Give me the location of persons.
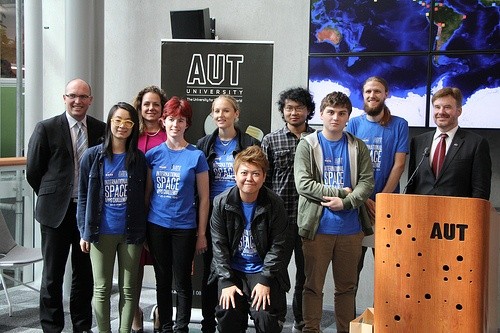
[261,88,316,333]
[145,96,209,333]
[197,94,261,333]
[346,76,409,333]
[77,102,146,333]
[131,85,166,333]
[26,79,107,333]
[294,91,375,333]
[209,145,294,333]
[405,88,492,201]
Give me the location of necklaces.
[219,132,235,155]
[145,128,160,136]
[167,143,187,169]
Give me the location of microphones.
[402,146,430,194]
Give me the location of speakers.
[170,7,216,39]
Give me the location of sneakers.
[292,320,303,333]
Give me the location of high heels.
[130,311,144,333]
[153,306,160,333]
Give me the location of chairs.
[0,209,43,316]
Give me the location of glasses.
[111,119,134,129]
[284,105,306,112]
[65,94,89,98]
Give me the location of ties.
[432,133,448,180]
[75,122,88,198]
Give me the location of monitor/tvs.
[307,0,500,131]
